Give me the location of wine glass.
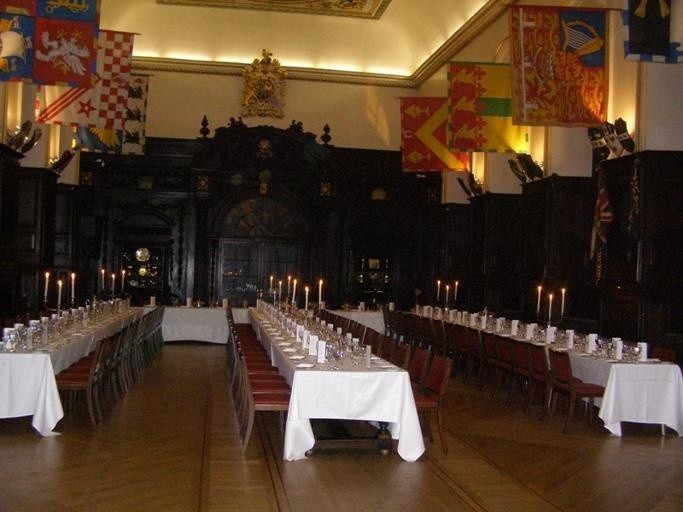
[3,297,131,353]
[268,300,367,370]
[412,304,652,362]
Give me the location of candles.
[99,269,125,293]
[435,279,458,305]
[268,275,323,311]
[40,272,76,307]
[536,286,564,320]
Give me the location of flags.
[445,59,532,156]
[507,3,611,130]
[70,73,150,157]
[399,96,472,174]
[625,171,643,240]
[31,30,134,132]
[1,0,102,89]
[586,175,616,259]
[619,0,683,64]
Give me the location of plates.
[34,307,142,354]
[250,306,400,370]
[413,309,615,364]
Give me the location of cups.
[150,297,394,311]
